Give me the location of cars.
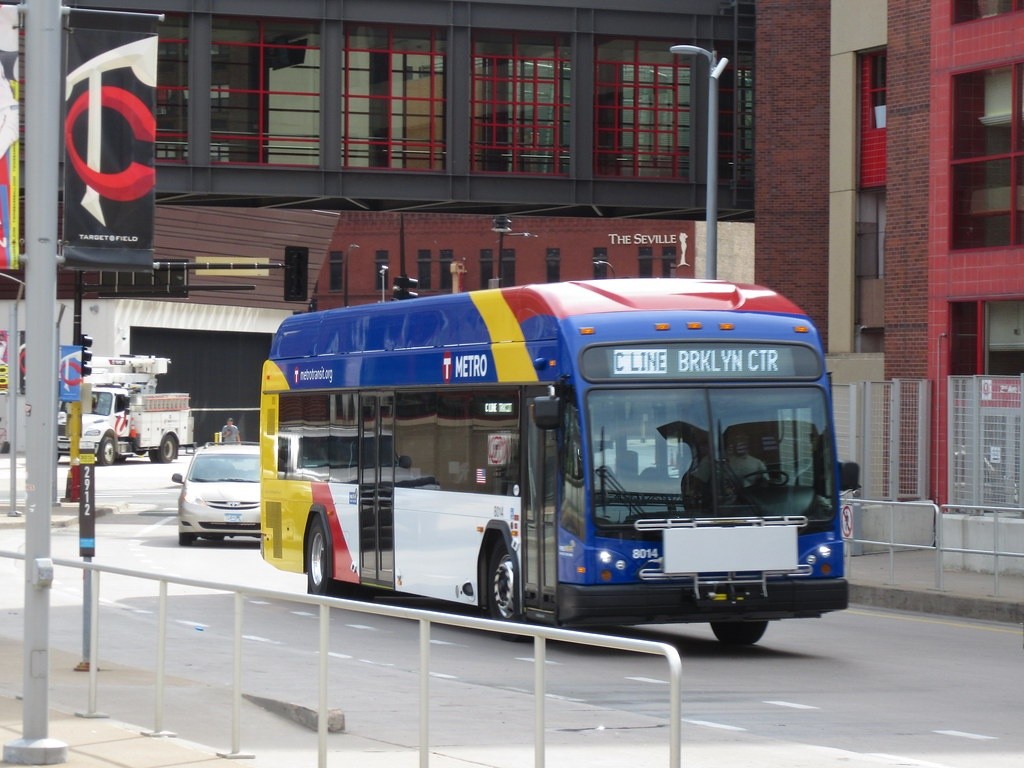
[171,441,261,546]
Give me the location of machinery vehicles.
[58,355,195,466]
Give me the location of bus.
[258,283,864,646]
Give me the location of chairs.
[627,450,656,479]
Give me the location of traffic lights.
[392,276,420,300]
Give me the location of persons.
[220,418,241,445]
[719,434,770,504]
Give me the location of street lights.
[491,216,514,288]
[669,40,729,279]
[592,259,617,279]
